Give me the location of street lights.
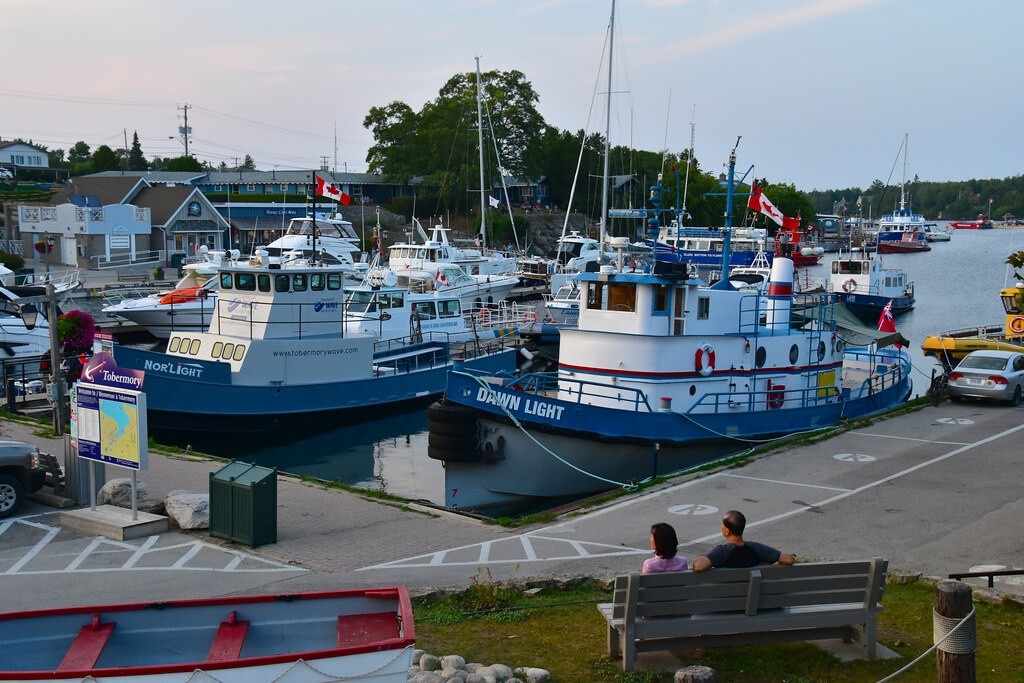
[361,200,368,253]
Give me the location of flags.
[747,181,799,231]
[314,176,351,208]
[436,271,449,287]
[489,195,499,209]
[877,300,901,349]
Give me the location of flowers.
[57,309,96,349]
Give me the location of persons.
[693,510,796,572]
[529,199,536,211]
[521,196,529,213]
[536,200,540,214]
[501,204,508,215]
[642,522,688,574]
[629,258,642,273]
[503,243,513,258]
[308,252,319,266]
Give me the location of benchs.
[373,347,444,376]
[595,557,889,672]
[115,266,149,282]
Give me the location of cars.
[946,350,1024,406]
[0,439,47,518]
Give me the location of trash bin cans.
[171,253,186,268]
[63,433,106,509]
[209,459,278,551]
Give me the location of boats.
[0,1,952,517]
[920,234,1024,375]
[948,198,995,230]
[0,582,418,683]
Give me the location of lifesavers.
[479,308,491,322]
[695,343,715,377]
[1009,316,1024,334]
[841,278,857,293]
[428,401,482,463]
